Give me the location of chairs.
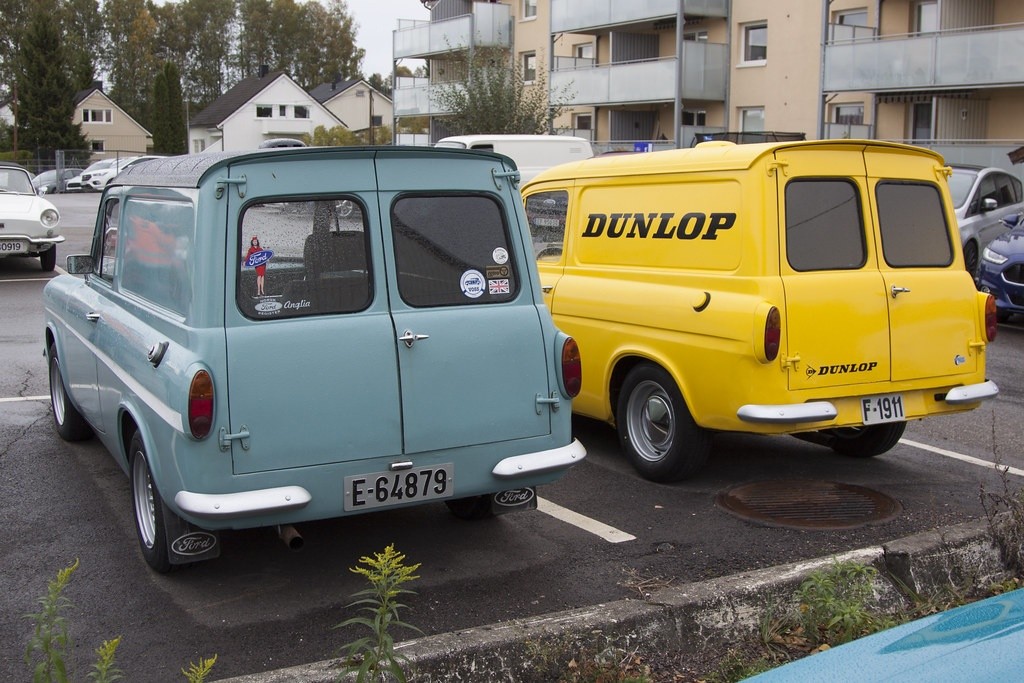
[303,229,367,274]
[282,277,369,319]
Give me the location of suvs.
[40,137,589,577]
[526,141,1000,483]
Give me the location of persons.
[131,212,190,299]
[247,238,267,297]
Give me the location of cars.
[0,161,65,271]
[91,154,168,193]
[975,212,1024,331]
[31,167,86,196]
[65,156,129,192]
[944,161,1024,277]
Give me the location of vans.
[432,133,596,195]
